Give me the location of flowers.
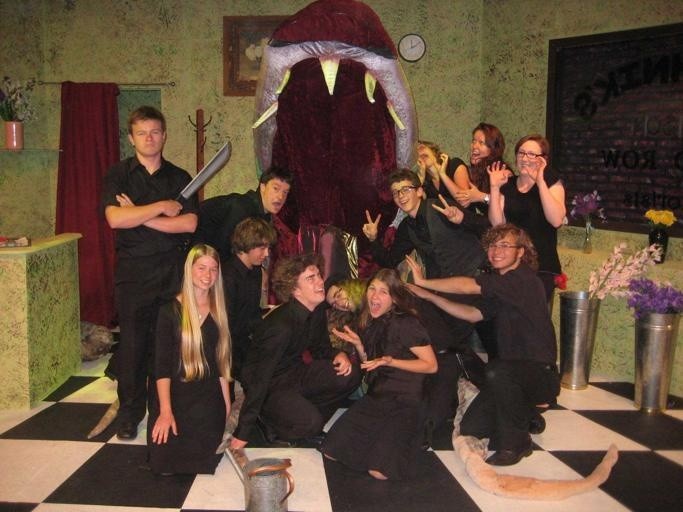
[644,206,676,225]
[586,243,662,303]
[567,190,609,240]
[553,272,567,294]
[622,274,681,314]
[0,75,37,121]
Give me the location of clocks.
[395,33,427,63]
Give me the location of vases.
[5,119,24,151]
[580,218,592,254]
[649,224,668,263]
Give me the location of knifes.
[174,141,232,205]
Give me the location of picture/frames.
[221,14,293,98]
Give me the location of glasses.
[391,185,417,197]
[489,243,520,251]
[516,151,544,159]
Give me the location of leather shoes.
[485,448,532,465]
[117,422,137,438]
[529,414,545,434]
[256,415,295,448]
